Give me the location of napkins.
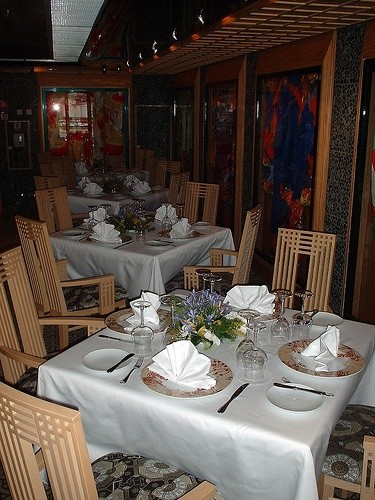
[127,289,161,331]
[154,203,176,227]
[77,177,91,188]
[301,325,341,374]
[83,183,105,197]
[90,221,122,245]
[224,283,277,314]
[147,341,217,392]
[134,181,150,195]
[124,175,141,186]
[83,208,110,223]
[169,217,192,239]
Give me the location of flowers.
[168,291,250,350]
[108,209,150,233]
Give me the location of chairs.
[0,148,375,500]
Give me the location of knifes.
[273,382,334,398]
[107,353,135,373]
[113,241,134,250]
[217,383,249,414]
[120,356,144,385]
[99,334,133,343]
[154,239,174,244]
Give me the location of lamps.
[117,63,121,72]
[101,64,107,74]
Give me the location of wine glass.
[176,203,186,222]
[195,268,212,291]
[131,301,154,358]
[243,322,269,381]
[159,294,183,347]
[270,289,293,346]
[87,197,147,241]
[235,309,260,379]
[290,290,313,340]
[160,203,172,231]
[202,273,222,293]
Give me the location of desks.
[77,169,141,184]
[33,289,375,500]
[66,183,169,218]
[49,213,237,300]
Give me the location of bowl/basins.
[193,221,211,230]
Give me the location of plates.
[104,307,172,335]
[277,338,365,378]
[305,312,344,327]
[80,348,133,372]
[265,383,324,413]
[140,356,234,400]
[144,240,171,248]
[62,227,85,239]
